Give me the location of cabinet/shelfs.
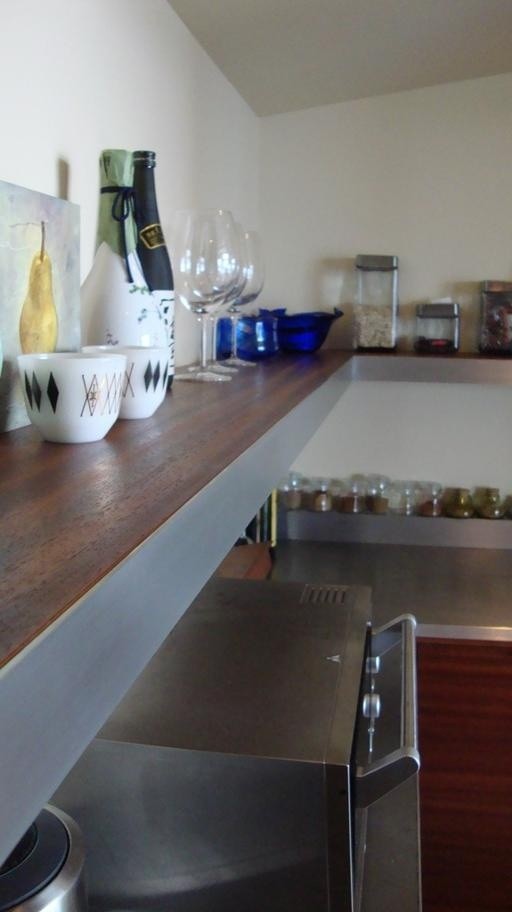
[353,340,510,394]
[0,349,354,872]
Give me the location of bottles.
[351,254,512,356]
[280,474,511,518]
[80,149,174,396]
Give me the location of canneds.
[478,280,512,356]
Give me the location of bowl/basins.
[275,308,345,352]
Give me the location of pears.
[19,222,59,354]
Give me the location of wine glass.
[178,212,266,384]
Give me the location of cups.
[17,344,171,443]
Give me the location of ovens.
[54,575,418,910]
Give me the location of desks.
[267,507,511,646]
